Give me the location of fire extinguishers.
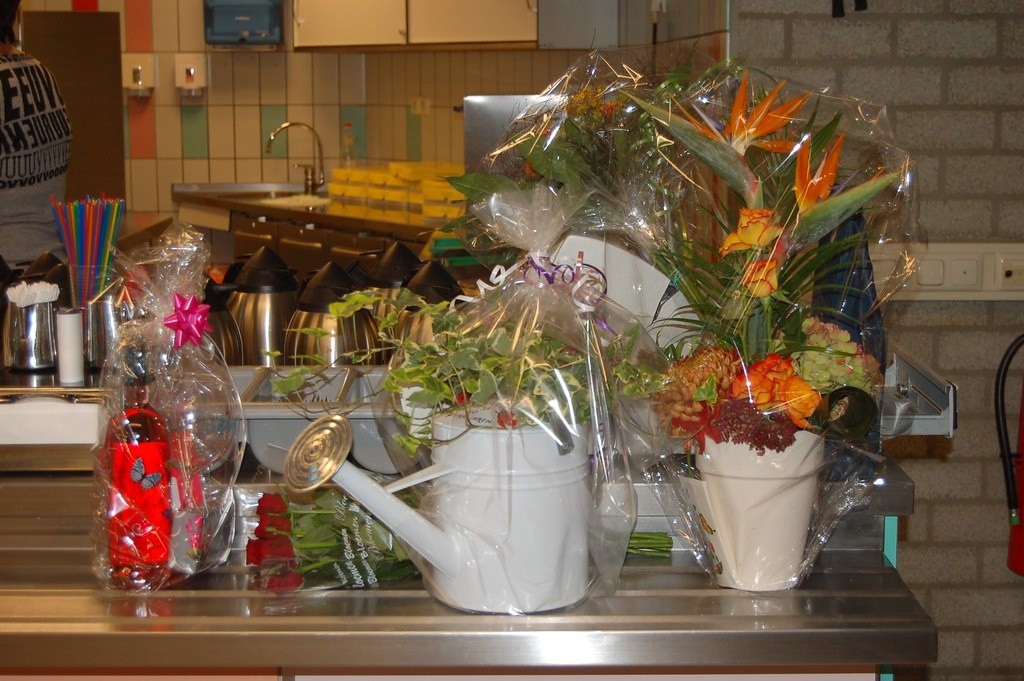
[994,331,1024,574]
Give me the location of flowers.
[242,491,673,591]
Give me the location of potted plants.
[458,43,923,594]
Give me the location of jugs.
[0,237,468,384]
[281,394,590,613]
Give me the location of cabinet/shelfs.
[290,0,540,50]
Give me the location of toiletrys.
[172,52,212,108]
[121,51,159,108]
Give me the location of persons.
[0,0,73,323]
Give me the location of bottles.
[103,325,174,588]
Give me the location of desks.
[0,472,939,680]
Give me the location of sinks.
[218,188,319,203]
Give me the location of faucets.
[266,120,326,196]
